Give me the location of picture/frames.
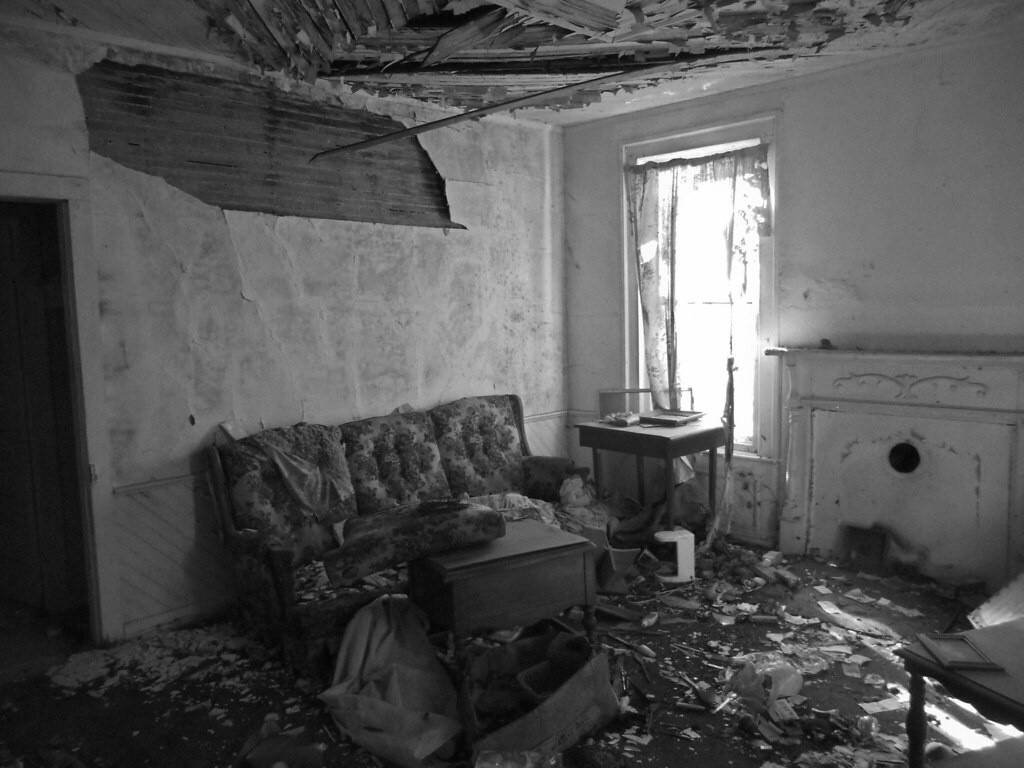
[915,632,1003,671]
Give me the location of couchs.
[203,394,588,663]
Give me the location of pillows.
[339,494,508,587]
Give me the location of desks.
[895,617,1024,768]
[577,418,726,527]
[408,519,601,717]
